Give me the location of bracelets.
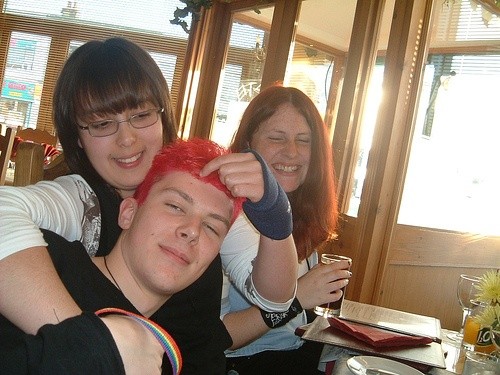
[260,296,303,329]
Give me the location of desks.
[294,314,500,375]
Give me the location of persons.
[0,37,352,375]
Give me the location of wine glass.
[448,274,485,343]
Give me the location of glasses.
[75,109,164,137]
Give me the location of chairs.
[0,125,61,187]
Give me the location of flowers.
[476,269,500,331]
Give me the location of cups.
[461,351,500,375]
[461,301,496,363]
[310,253,353,318]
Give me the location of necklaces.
[104,256,121,290]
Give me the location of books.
[294,298,449,374]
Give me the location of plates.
[345,356,424,375]
